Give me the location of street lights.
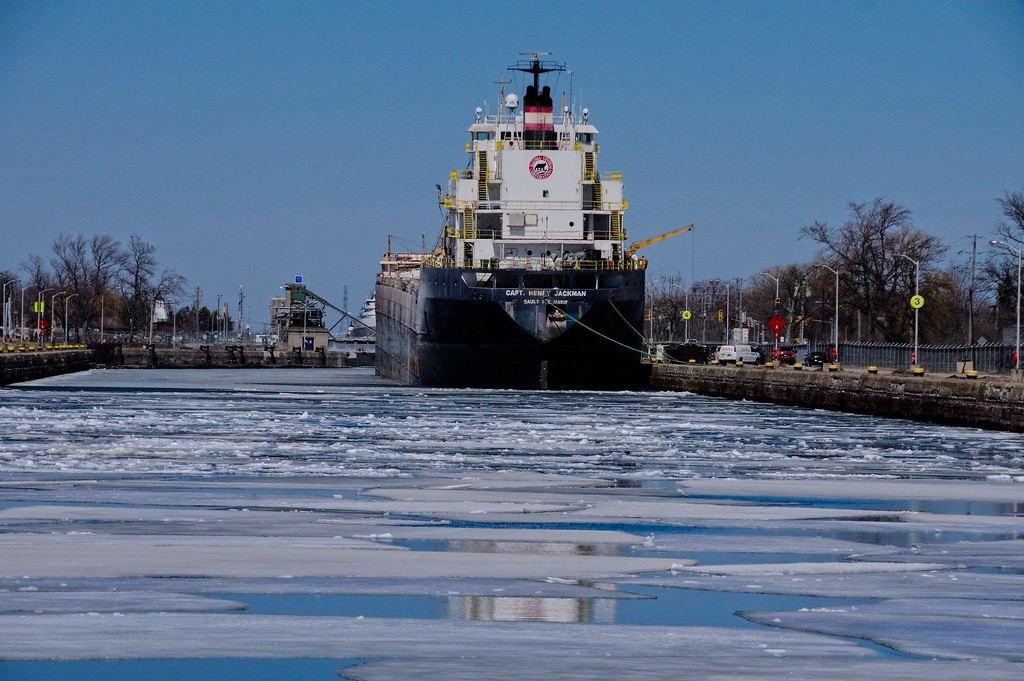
[989,239,1021,369]
[756,272,778,349]
[21,284,38,342]
[3,279,21,343]
[38,289,54,343]
[216,294,223,335]
[670,284,687,339]
[891,254,919,365]
[815,264,838,363]
[101,287,121,340]
[65,294,80,342]
[710,280,729,345]
[51,292,66,345]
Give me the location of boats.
[375,52,695,392]
[346,290,376,337]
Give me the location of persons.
[110,332,155,342]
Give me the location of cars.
[805,352,829,367]
[680,339,700,346]
[0,326,182,342]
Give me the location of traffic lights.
[766,314,788,338]
[39,319,49,330]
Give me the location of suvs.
[771,346,795,365]
[713,345,765,365]
